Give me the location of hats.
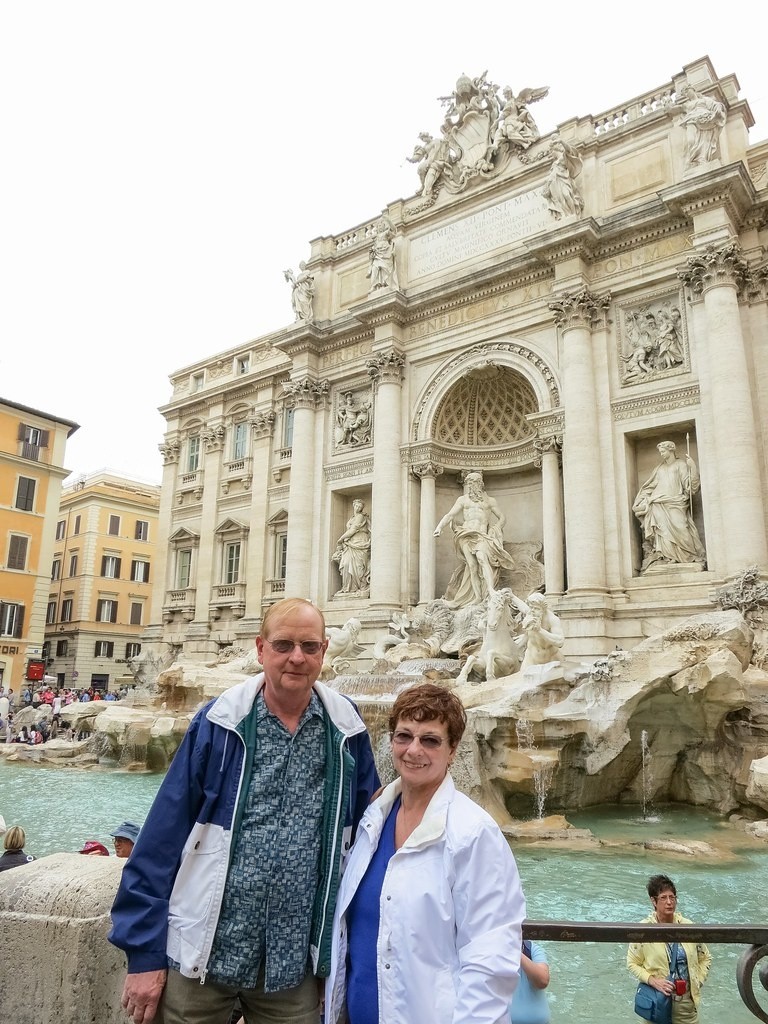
[109,821,141,844]
[79,841,109,857]
[35,689,43,693]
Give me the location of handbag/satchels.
[44,731,50,736]
[635,982,672,1024]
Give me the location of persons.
[0,712,72,745]
[491,86,531,148]
[409,131,448,196]
[500,587,564,670]
[337,392,371,447]
[0,684,121,716]
[544,139,584,220]
[366,216,399,293]
[664,84,727,170]
[434,471,506,604]
[76,840,109,856]
[110,821,140,857]
[106,597,381,1024]
[324,683,525,1024]
[337,499,371,593]
[620,312,684,385]
[626,874,712,1024]
[633,440,704,568]
[0,825,37,872]
[291,261,315,319]
[322,618,362,683]
[509,940,551,1024]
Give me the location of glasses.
[389,731,450,749]
[112,838,129,845]
[267,639,325,654]
[654,894,677,901]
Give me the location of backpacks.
[34,732,43,742]
